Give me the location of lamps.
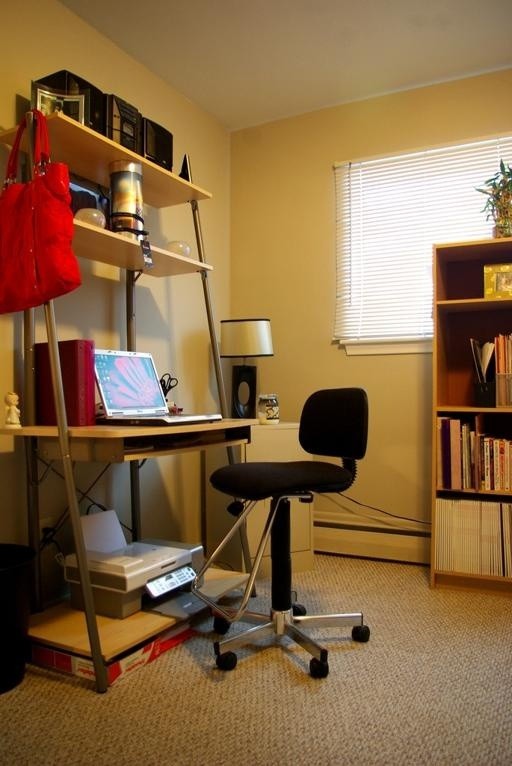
[220,318,274,418]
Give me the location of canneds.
[257,394,280,425]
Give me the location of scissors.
[160,373,178,397]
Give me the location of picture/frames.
[483,263,512,298]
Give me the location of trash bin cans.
[0,543,34,695]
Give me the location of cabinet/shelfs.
[429,239,512,598]
[2,109,257,694]
[204,418,315,584]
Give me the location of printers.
[64,538,218,620]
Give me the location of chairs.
[209,388,370,678]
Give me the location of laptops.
[94,349,222,425]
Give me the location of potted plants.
[472,159,512,237]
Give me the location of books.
[433,330,511,582]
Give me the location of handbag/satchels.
[0,108,81,315]
